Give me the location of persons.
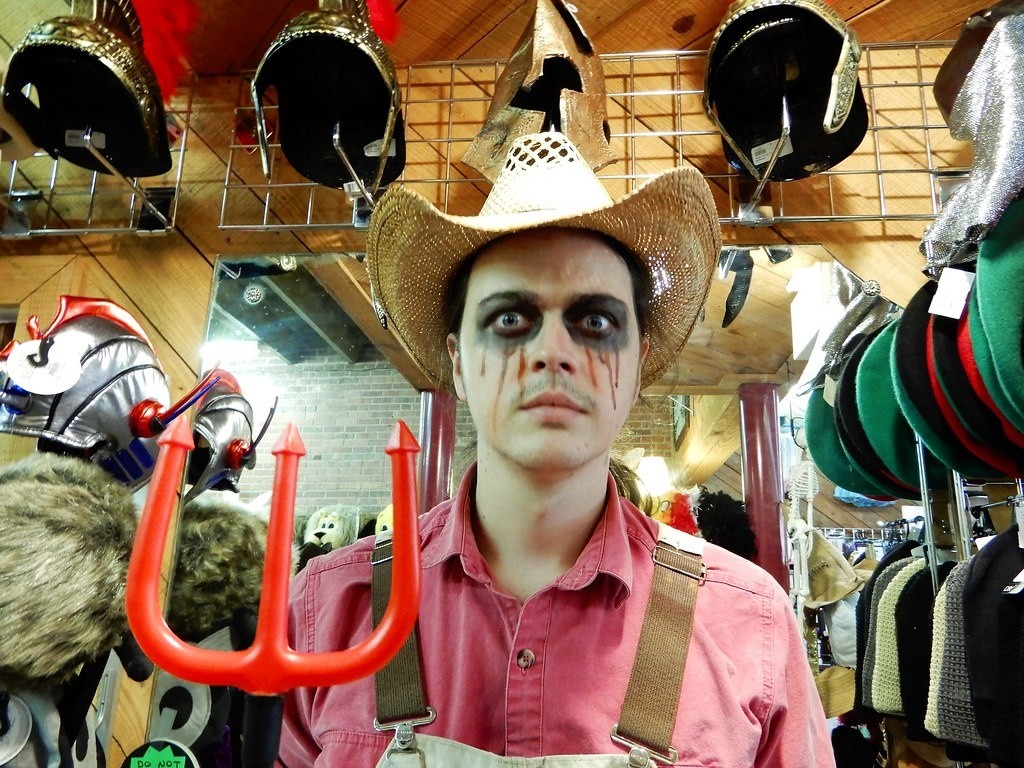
[274,133,837,767]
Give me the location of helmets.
[700,0,869,186]
[1,0,172,178]
[251,0,407,188]
[0,295,222,497]
[183,368,278,507]
[459,0,618,187]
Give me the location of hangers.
[886,515,925,547]
[970,506,995,534]
[854,531,878,544]
[1006,496,1017,524]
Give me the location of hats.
[786,201,1024,768]
[365,132,723,392]
[921,0,1024,274]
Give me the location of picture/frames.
[672,396,689,451]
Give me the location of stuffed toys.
[293,505,395,570]
